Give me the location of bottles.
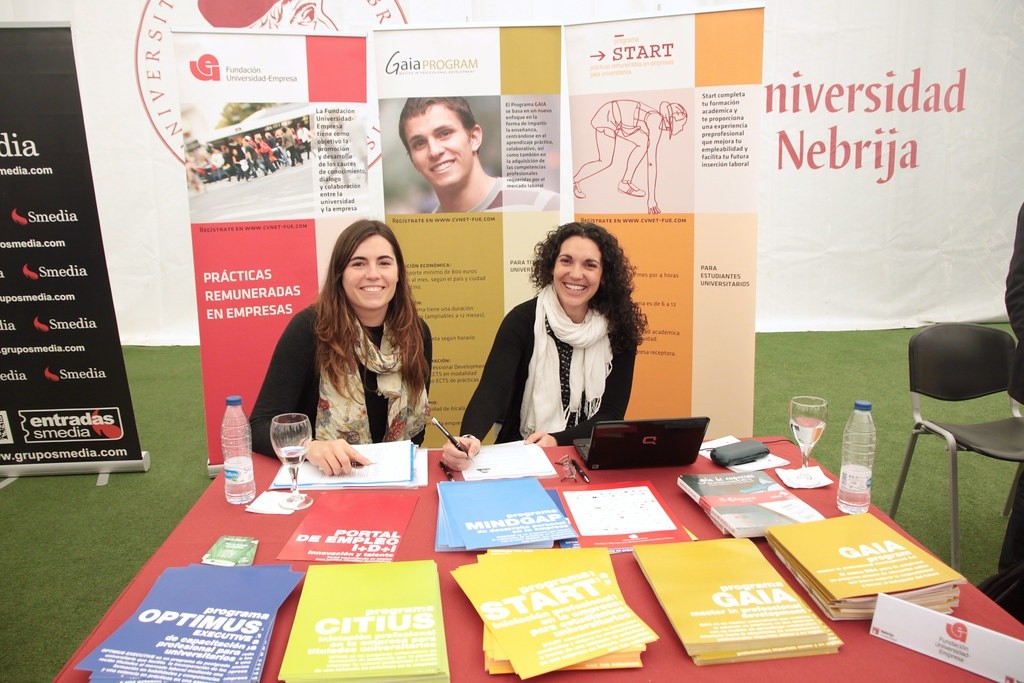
[836,400,876,514]
[220,395,256,505]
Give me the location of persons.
[399,96,561,213]
[442,221,647,471]
[186,123,310,193]
[246,219,432,474]
[997,203,1024,572]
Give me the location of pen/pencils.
[438,459,455,481]
[352,461,378,467]
[430,417,476,465]
[571,458,590,483]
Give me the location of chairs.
[889,323,1024,573]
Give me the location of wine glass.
[787,396,828,486]
[269,413,314,511]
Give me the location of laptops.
[572,416,710,470]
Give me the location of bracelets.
[301,437,309,446]
[462,434,476,439]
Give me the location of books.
[676,470,825,538]
[72,477,846,683]
[269,441,429,491]
[764,513,966,621]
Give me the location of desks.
[53,435,1024,683]
